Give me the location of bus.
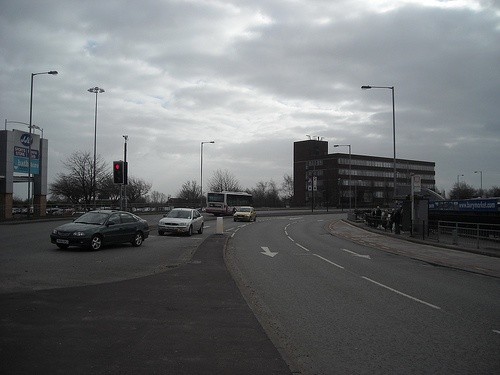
[204,191,252,217]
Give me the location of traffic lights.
[113,160,124,185]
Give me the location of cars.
[50,209,150,251]
[233,207,257,223]
[12,207,110,223]
[158,208,204,236]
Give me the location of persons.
[375,205,401,234]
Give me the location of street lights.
[201,141,215,212]
[457,174,464,188]
[122,134,129,212]
[88,87,105,205]
[26,70,59,220]
[474,170,483,190]
[333,144,352,209]
[361,84,397,209]
